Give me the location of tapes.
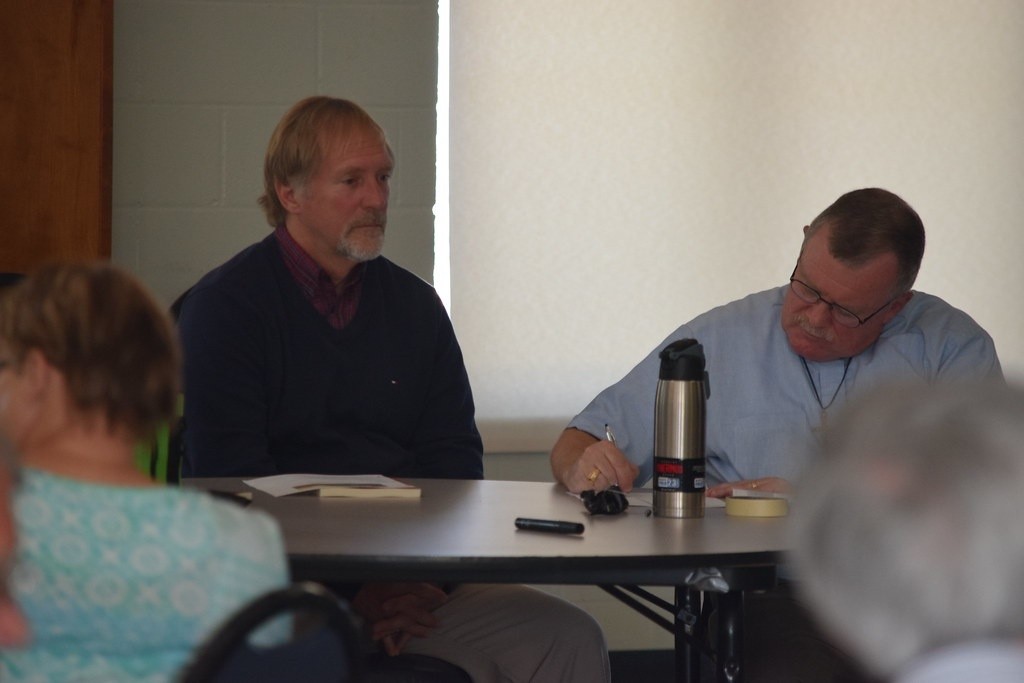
[727,496,789,518]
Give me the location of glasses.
[790,265,899,330]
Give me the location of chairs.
[180,580,362,683]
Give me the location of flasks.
[653,337,710,519]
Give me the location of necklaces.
[801,357,852,411]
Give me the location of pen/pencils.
[604,423,618,450]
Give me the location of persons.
[551,187,1006,683]
[0,261,293,683]
[151,95,611,683]
[783,373,1024,683]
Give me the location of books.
[319,475,421,498]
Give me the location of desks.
[173,476,807,683]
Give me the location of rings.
[587,468,600,482]
[752,482,757,488]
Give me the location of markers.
[514,517,585,535]
